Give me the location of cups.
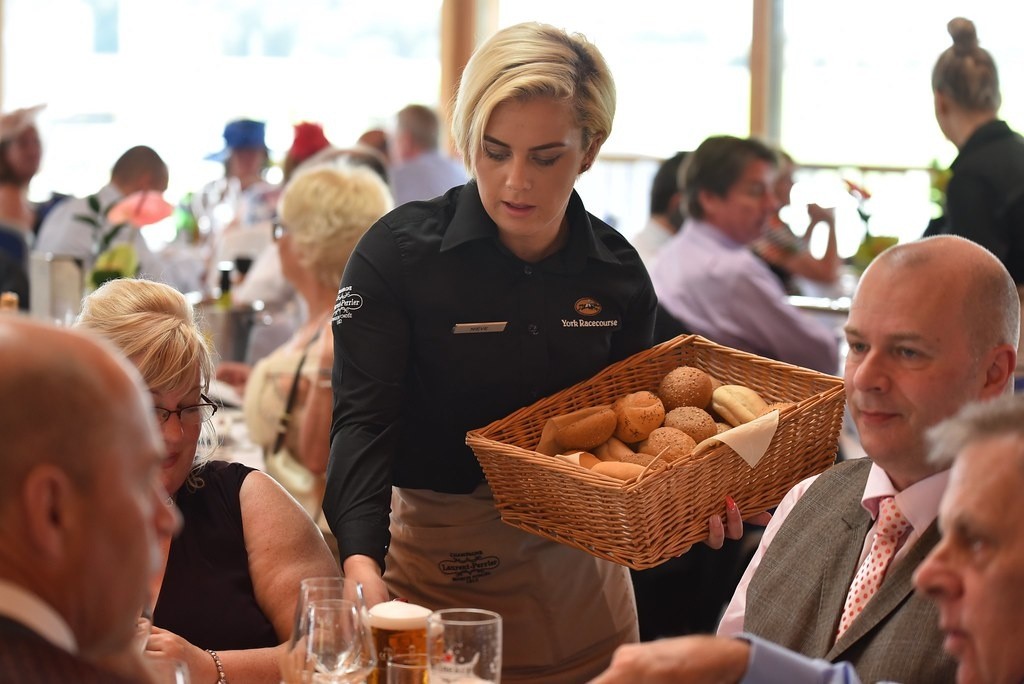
[294,599,377,684]
[367,602,435,684]
[139,657,189,684]
[279,577,371,683]
[386,654,429,684]
[428,608,502,684]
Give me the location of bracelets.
[206,649,225,684]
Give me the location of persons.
[0,316,183,684]
[631,135,838,377]
[319,20,771,684]
[588,392,1024,684]
[78,279,340,684]
[0,104,470,565]
[718,234,1024,684]
[924,18,1024,379]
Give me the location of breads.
[555,366,797,480]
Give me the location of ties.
[834,493,914,646]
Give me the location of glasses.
[272,219,294,242]
[152,394,219,427]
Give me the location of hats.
[205,119,265,160]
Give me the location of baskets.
[465,333,847,570]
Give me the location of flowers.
[73,184,171,294]
